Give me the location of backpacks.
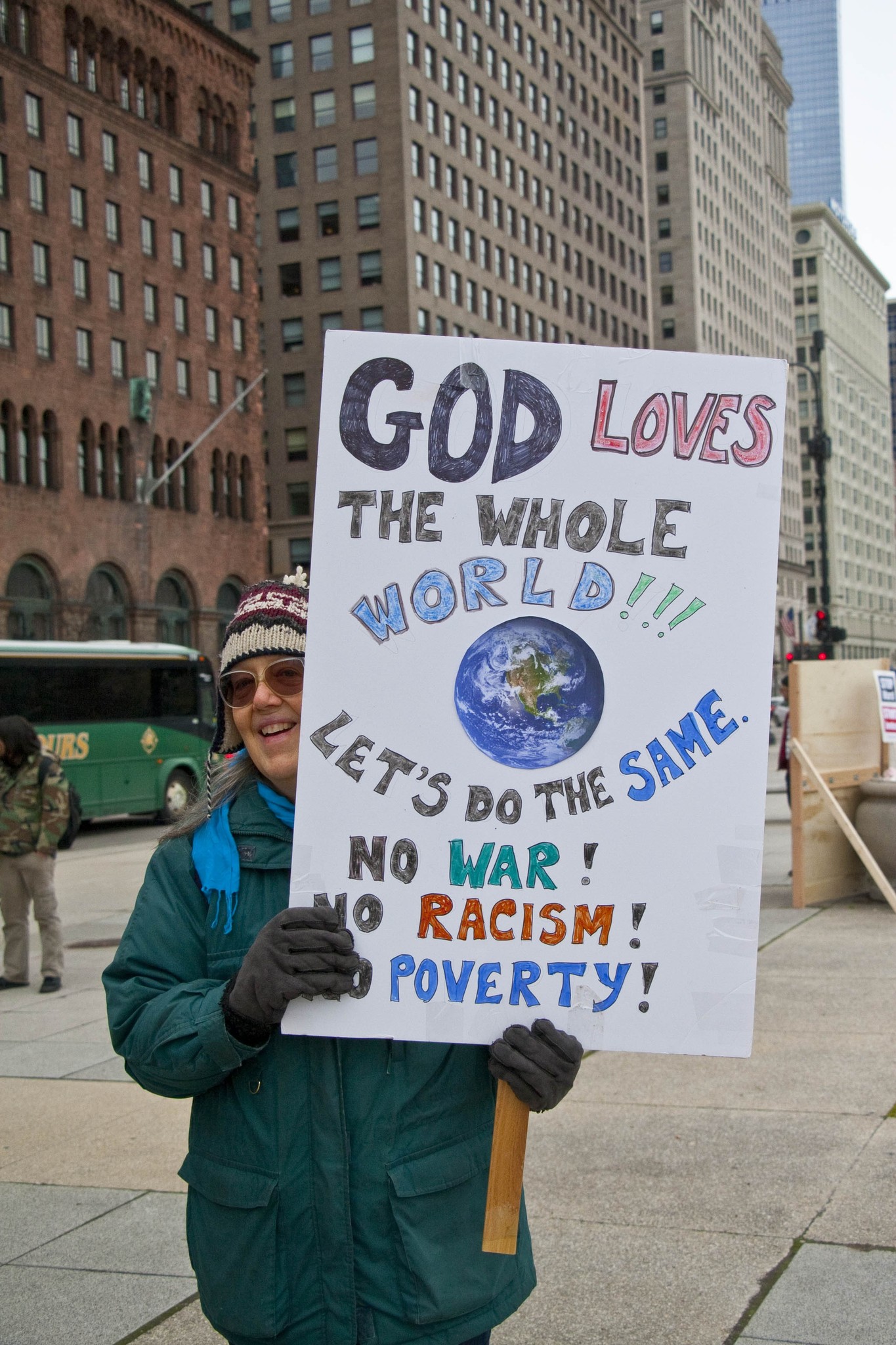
[36,758,82,850]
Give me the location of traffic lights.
[786,608,833,661]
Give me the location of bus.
[0,635,218,827]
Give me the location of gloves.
[228,906,362,1025]
[490,1018,584,1113]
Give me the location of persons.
[0,716,81,996]
[100,566,583,1345]
[777,675,793,878]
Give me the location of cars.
[770,696,790,727]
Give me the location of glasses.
[217,657,306,709]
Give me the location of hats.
[206,566,310,818]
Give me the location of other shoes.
[0,976,29,990]
[40,976,61,991]
